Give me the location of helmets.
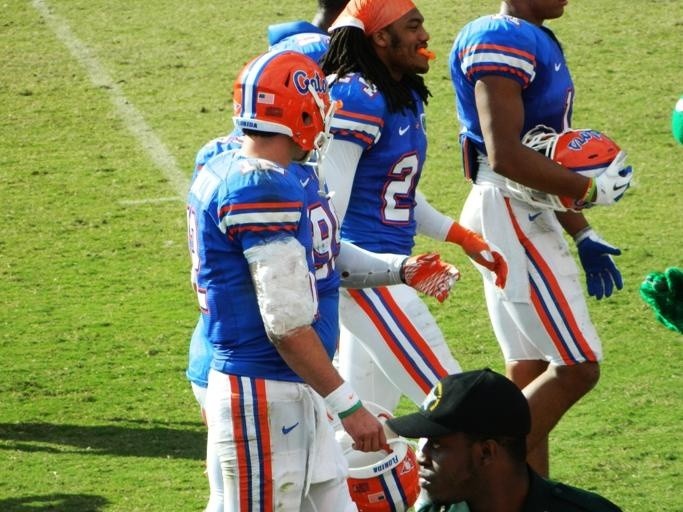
[230,48,337,167]
[507,126,624,213]
[339,432,420,511]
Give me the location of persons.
[189,0,508,511]
[386,367,623,512]
[452,1,632,478]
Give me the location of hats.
[388,367,532,440]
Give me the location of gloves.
[445,223,508,289]
[574,231,624,298]
[401,252,460,302]
[588,148,632,206]
